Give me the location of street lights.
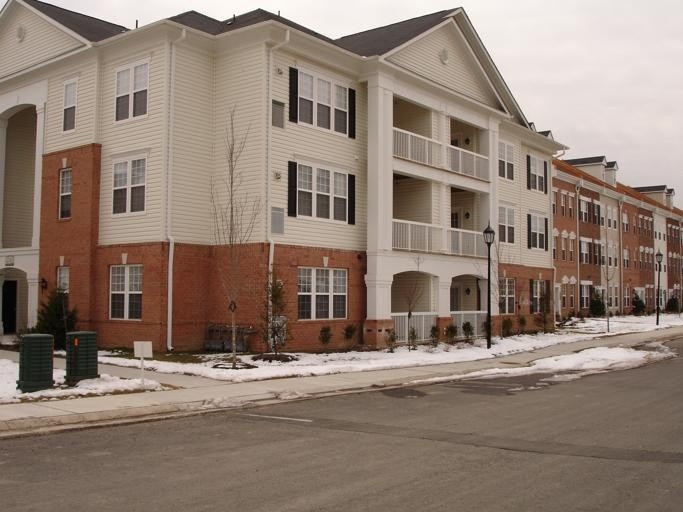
[479,219,498,351]
[653,247,665,325]
